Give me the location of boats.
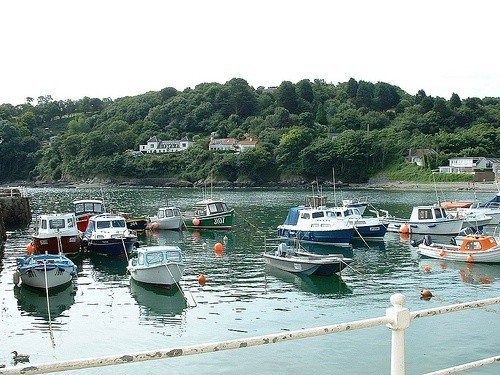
[126,246,187,286]
[263,232,353,277]
[30,170,236,256]
[16,250,77,290]
[275,166,500,263]
